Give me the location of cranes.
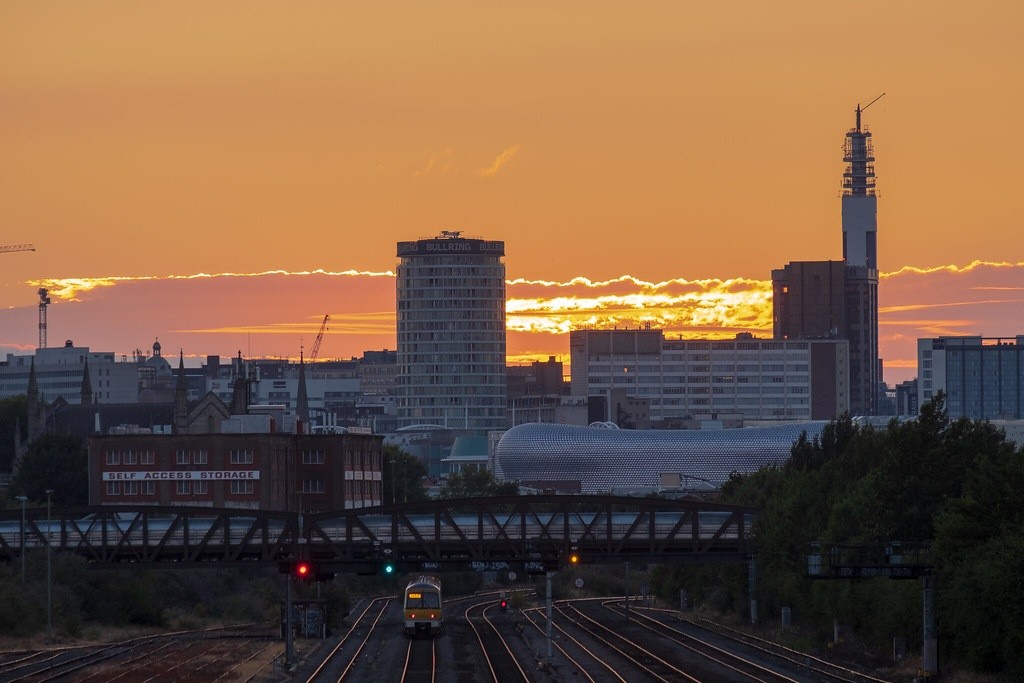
[37,287,50,349]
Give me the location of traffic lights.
[570,555,579,564]
[383,563,395,575]
[296,563,309,575]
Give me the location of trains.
[403,576,444,638]
